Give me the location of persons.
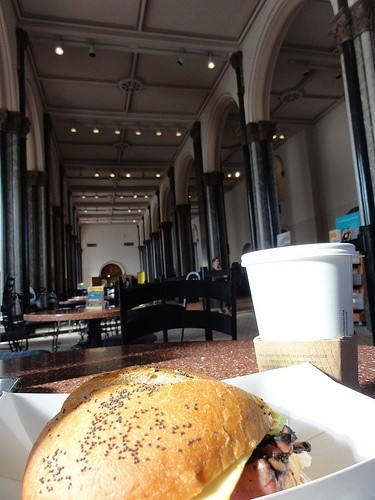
[211,256,233,317]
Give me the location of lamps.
[94,169,161,178]
[82,192,149,198]
[303,65,308,76]
[207,52,215,69]
[55,40,65,55]
[177,49,184,65]
[71,127,182,137]
[89,39,96,58]
[336,68,342,79]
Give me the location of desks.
[22,309,120,350]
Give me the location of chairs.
[0,274,62,352]
[117,276,237,345]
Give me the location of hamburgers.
[21,365,312,500]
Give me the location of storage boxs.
[253,336,358,388]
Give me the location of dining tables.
[0,339,375,399]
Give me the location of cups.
[240,243,357,339]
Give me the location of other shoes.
[226,309,232,316]
[218,309,224,314]
